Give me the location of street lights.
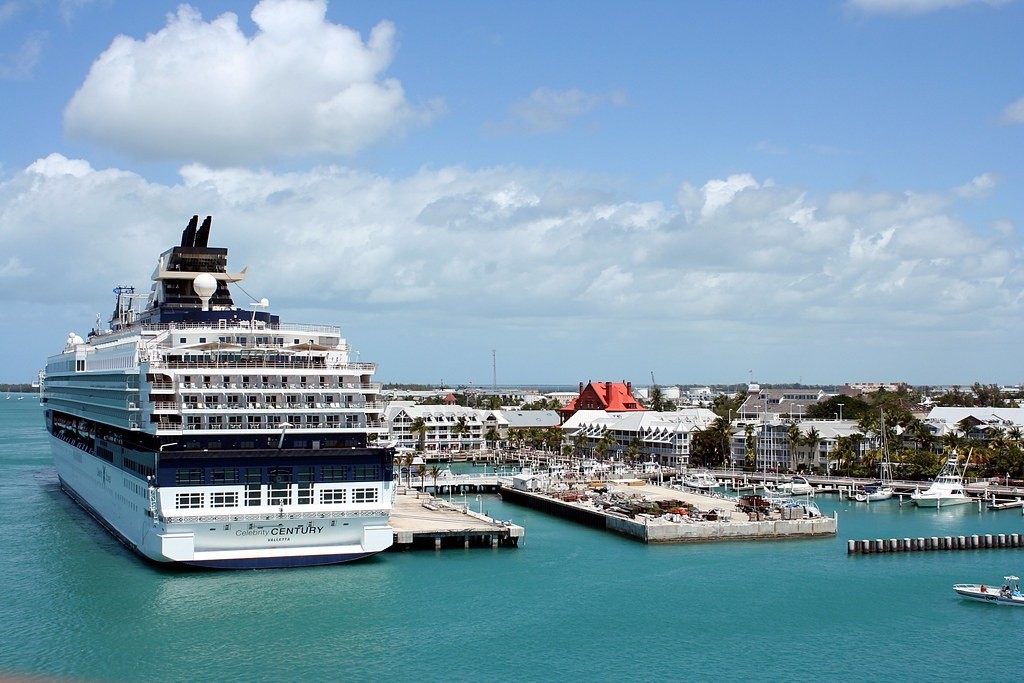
[650,453,656,462]
[834,413,838,419]
[617,449,623,461]
[790,402,795,419]
[754,406,761,420]
[729,409,732,424]
[838,404,844,420]
[476,493,483,516]
[445,485,453,505]
[459,489,467,508]
[797,404,804,421]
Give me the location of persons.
[981,585,986,592]
[1015,585,1019,591]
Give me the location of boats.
[909,447,972,507]
[683,473,722,488]
[775,474,813,495]
[952,575,1024,608]
[849,486,895,501]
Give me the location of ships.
[37,215,404,573]
